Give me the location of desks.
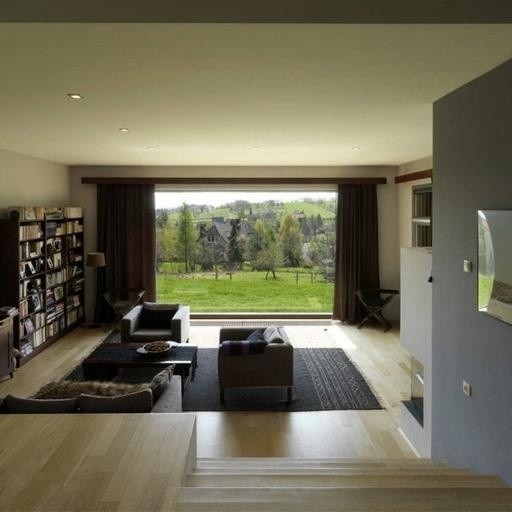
[354,288,399,334]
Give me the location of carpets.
[66,345,385,412]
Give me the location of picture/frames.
[476,208,512,327]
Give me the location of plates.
[135,340,179,359]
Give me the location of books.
[0,206,84,358]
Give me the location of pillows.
[6,364,174,411]
[246,329,265,342]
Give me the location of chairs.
[3,373,182,412]
[217,325,294,404]
[101,290,146,332]
[121,301,191,345]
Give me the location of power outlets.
[461,380,472,398]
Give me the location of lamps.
[87,253,106,329]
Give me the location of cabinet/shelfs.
[0,311,21,384]
[2,203,87,365]
